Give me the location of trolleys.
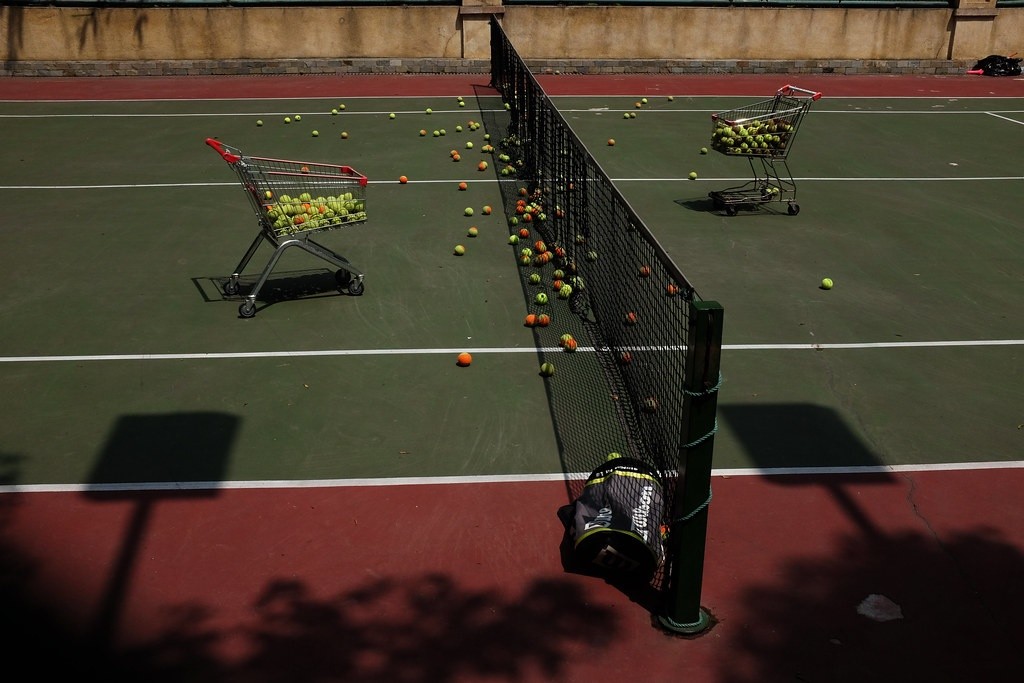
[705,85,824,215]
[207,137,371,318]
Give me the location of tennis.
[256,93,794,376]
[606,452,622,460]
[820,277,833,289]
